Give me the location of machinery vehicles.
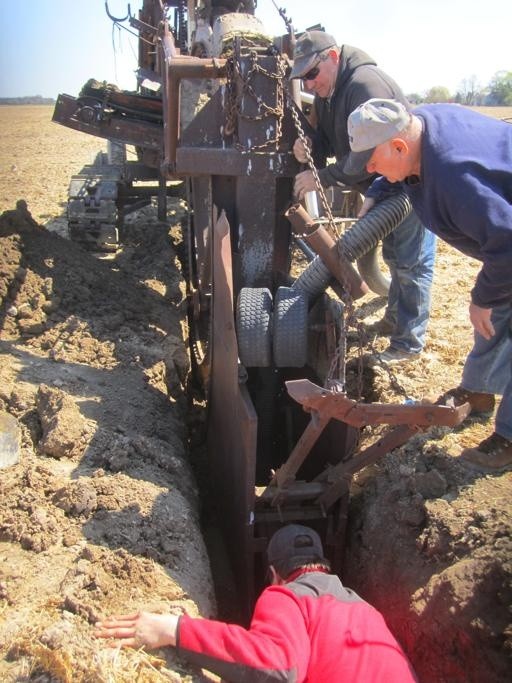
[46,0,416,629]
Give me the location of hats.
[289,31,337,80]
[343,98,408,176]
[267,525,323,562]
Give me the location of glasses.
[300,61,320,81]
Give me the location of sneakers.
[462,432,512,473]
[371,346,421,364]
[433,387,495,418]
[365,320,392,338]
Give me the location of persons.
[340,94,512,473]
[289,26,437,373]
[88,522,426,683]
